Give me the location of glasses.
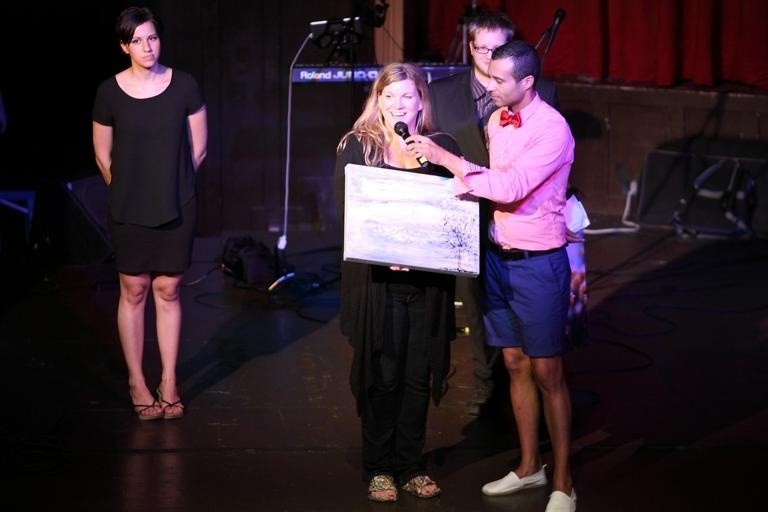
[473,43,496,55]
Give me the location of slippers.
[368,474,398,503]
[401,474,441,498]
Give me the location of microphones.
[393,117,428,169]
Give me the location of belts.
[490,243,565,261]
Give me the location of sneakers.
[544,488,578,512]
[480,467,549,497]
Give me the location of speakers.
[636,134,768,238]
[44,172,126,290]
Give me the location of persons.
[332,60,468,505]
[428,9,560,435]
[90,8,212,424]
[402,36,587,512]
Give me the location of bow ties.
[498,110,522,128]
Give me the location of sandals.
[130,390,163,420]
[156,387,185,419]
